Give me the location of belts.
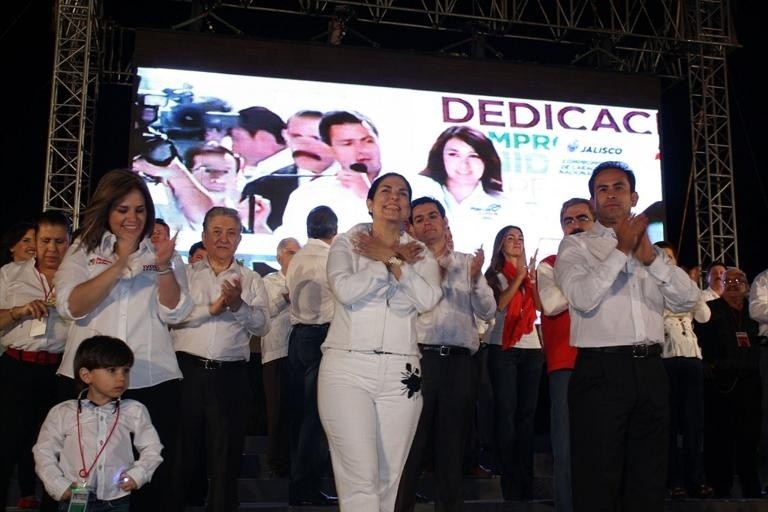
[6,347,57,366]
[198,358,245,370]
[418,346,469,356]
[577,344,659,358]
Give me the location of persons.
[134,106,512,259]
[554,158,704,509]
[0,161,768,510]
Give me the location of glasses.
[565,217,593,225]
[723,278,745,285]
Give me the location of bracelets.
[646,247,657,266]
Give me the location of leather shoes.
[288,486,338,506]
